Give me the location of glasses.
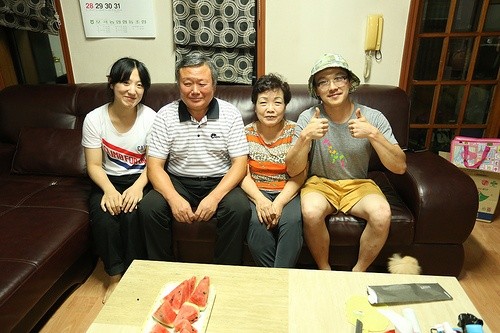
[314,74,350,90]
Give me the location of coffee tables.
[88,258,491,333]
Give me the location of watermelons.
[150,275,210,333]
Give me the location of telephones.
[365,15,383,50]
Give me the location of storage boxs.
[439,150,500,224]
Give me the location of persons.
[81,58,157,304]
[140,56,250,266]
[238,73,308,268]
[285,54,407,271]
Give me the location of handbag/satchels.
[450,136,500,173]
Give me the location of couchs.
[0,85,479,332]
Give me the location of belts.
[191,176,219,180]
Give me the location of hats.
[308,53,361,100]
